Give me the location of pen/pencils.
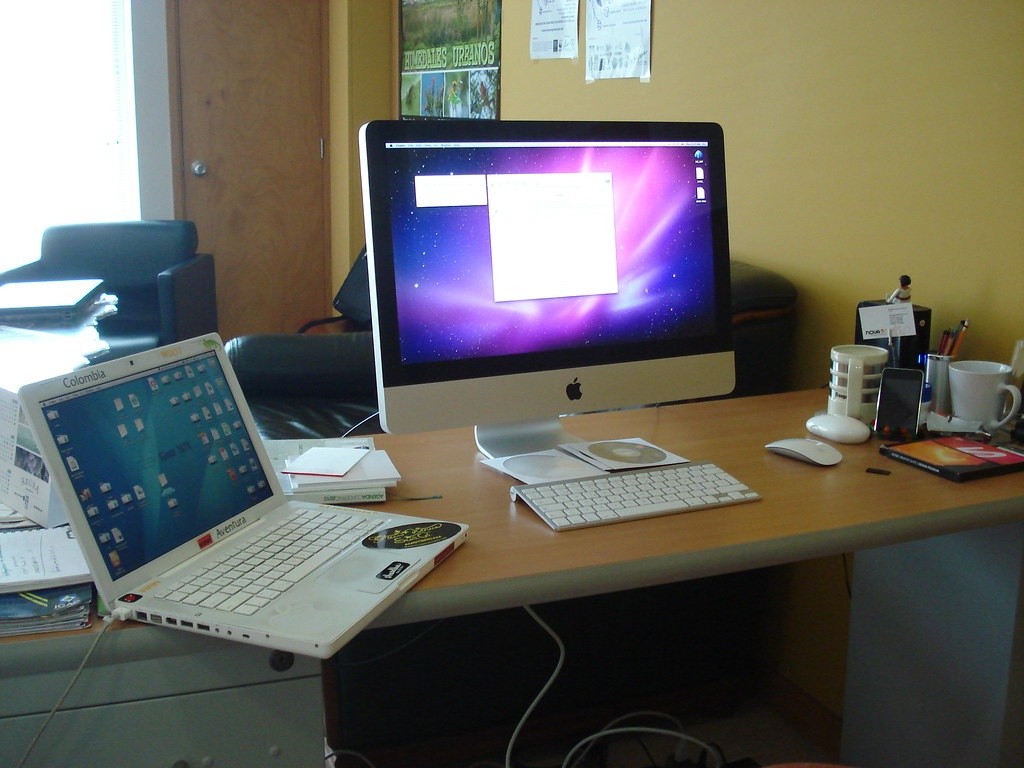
[936,318,971,357]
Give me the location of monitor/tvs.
[358,118,737,459]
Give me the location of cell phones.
[874,368,924,435]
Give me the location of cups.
[1003,340,1024,421]
[926,354,953,411]
[949,360,1021,437]
[827,344,888,431]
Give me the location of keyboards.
[511,462,763,531]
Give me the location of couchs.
[221,241,798,752]
[0,220,218,367]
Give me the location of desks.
[0,291,1024,768]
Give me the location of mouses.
[806,413,872,445]
[764,436,842,468]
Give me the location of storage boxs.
[0,385,69,530]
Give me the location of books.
[0,278,119,638]
[879,434,1024,484]
[261,437,402,504]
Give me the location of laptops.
[18,332,471,660]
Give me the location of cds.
[502,442,666,480]
